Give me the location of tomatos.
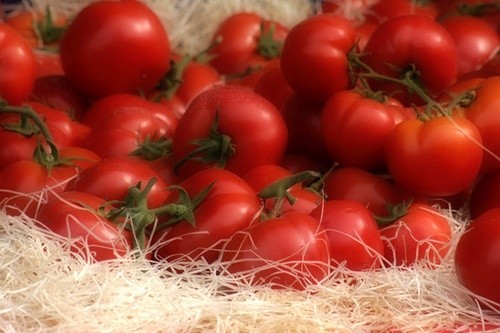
[0,0,500,312]
